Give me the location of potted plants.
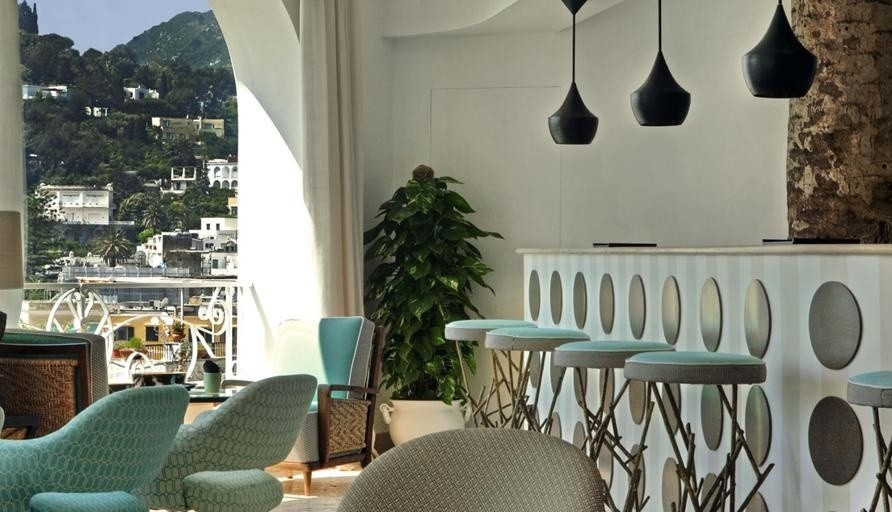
[362,166,505,446]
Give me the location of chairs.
[336,427,604,512]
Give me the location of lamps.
[547,1,817,145]
